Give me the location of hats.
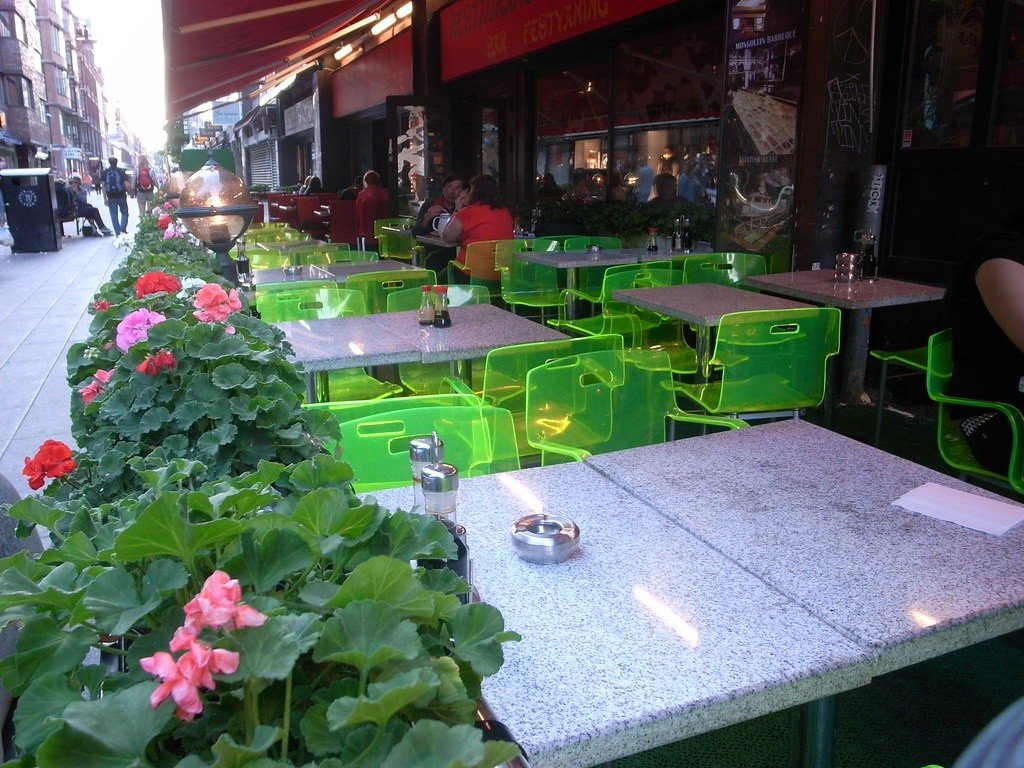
[70,177,82,186]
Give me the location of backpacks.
[104,167,124,197]
[138,164,155,192]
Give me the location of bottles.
[396,435,475,656]
[672,218,692,249]
[858,233,877,278]
[646,227,658,251]
[234,240,249,273]
[513,209,542,236]
[242,283,261,319]
[433,285,451,328]
[418,285,435,326]
[281,182,301,208]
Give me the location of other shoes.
[104,232,113,236]
[92,232,102,237]
[120,225,127,234]
[116,228,121,235]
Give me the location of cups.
[432,214,453,238]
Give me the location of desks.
[367,301,572,394]
[263,313,420,404]
[258,238,328,253]
[311,261,427,285]
[741,268,948,421]
[345,419,1024,768]
[249,264,336,288]
[610,283,818,438]
[380,224,418,268]
[414,235,461,260]
[513,250,637,320]
[603,248,711,264]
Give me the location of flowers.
[1,192,526,768]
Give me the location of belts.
[959,411,1000,437]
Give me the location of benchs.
[59,216,87,235]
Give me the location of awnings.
[161,0,391,124]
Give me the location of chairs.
[228,217,1024,767]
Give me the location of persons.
[132,154,160,215]
[54,179,76,217]
[298,175,325,195]
[411,173,470,269]
[66,173,112,236]
[540,173,564,198]
[356,169,389,202]
[94,173,101,195]
[340,176,364,201]
[436,175,514,290]
[612,145,719,217]
[100,156,133,238]
[82,172,93,195]
[68,169,81,179]
[941,270,1024,477]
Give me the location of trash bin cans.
[0,168,62,254]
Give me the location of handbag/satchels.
[82,217,97,236]
